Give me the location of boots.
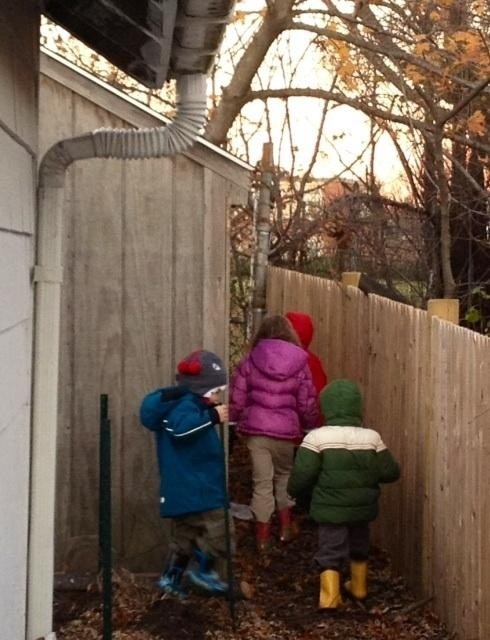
[319,561,372,612]
[255,504,294,556]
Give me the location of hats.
[174,349,228,399]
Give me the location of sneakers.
[154,568,230,602]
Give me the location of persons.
[230,311,328,550]
[140,349,230,599]
[287,379,400,609]
